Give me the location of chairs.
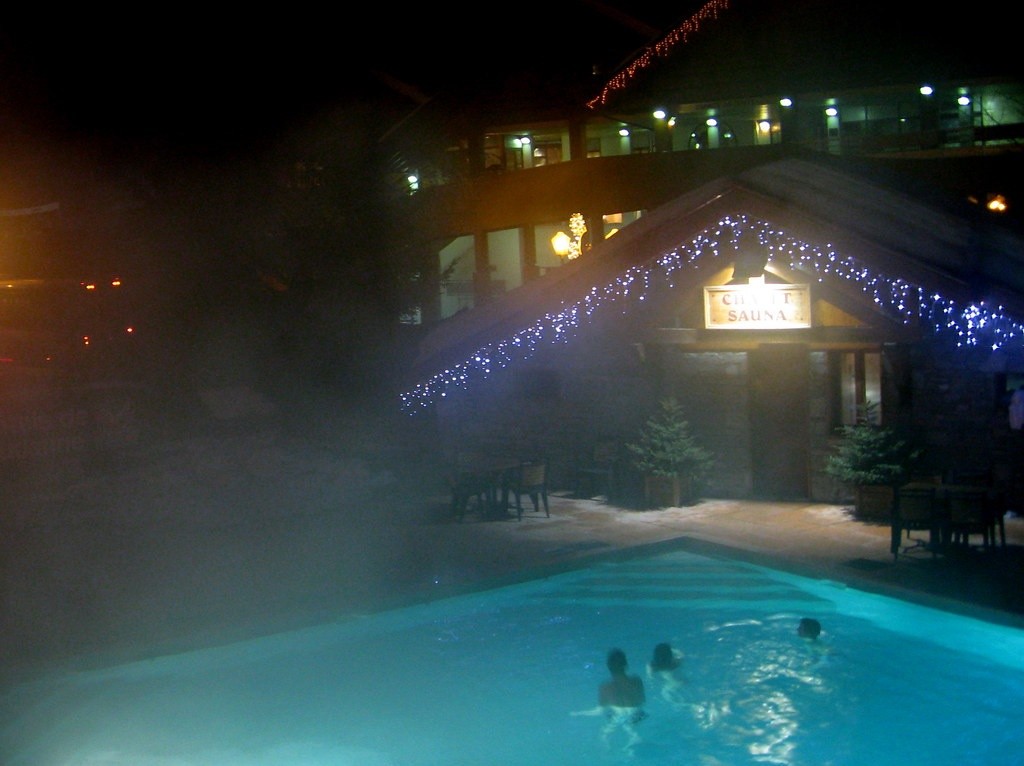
[450,458,550,522]
[889,473,1008,563]
[578,435,624,504]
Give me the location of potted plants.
[624,396,714,509]
[820,401,920,520]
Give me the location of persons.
[599,648,645,708]
[651,643,679,669]
[797,618,821,639]
